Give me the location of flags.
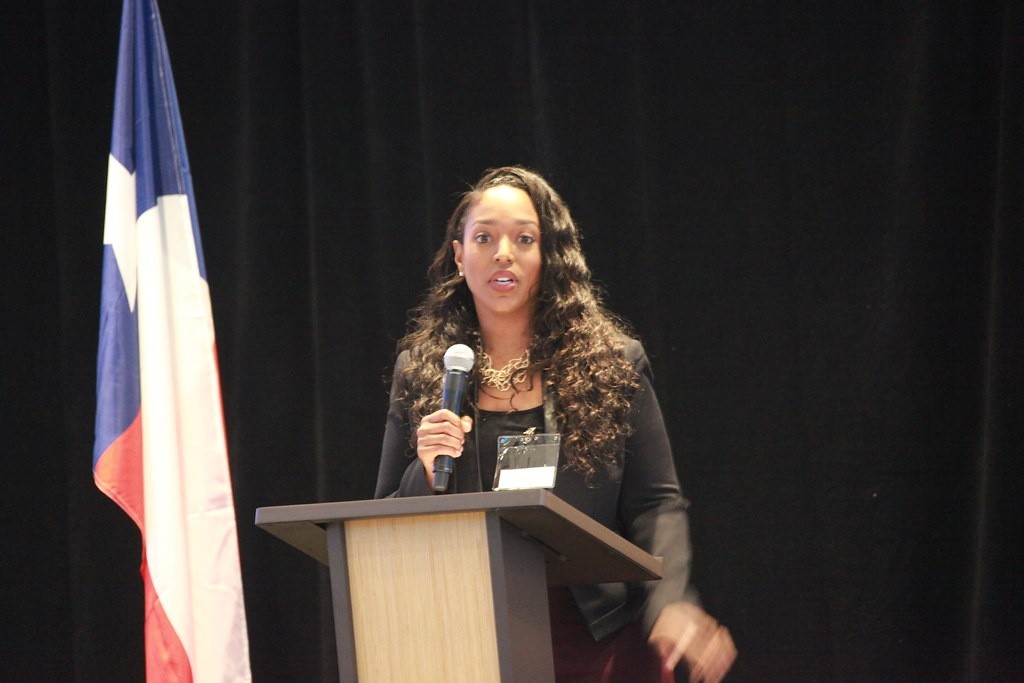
[93,0,252,682]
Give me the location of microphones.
[433,343,474,491]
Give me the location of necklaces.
[471,330,533,391]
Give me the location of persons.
[376,167,738,683]
